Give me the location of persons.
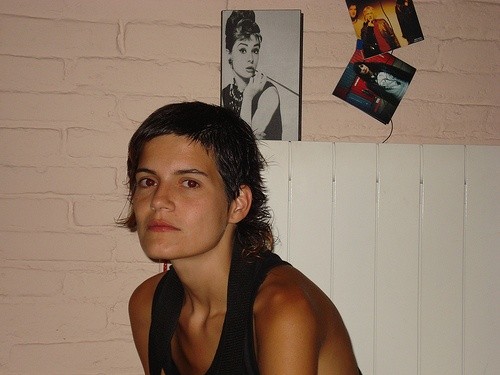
[220,10,283,141]
[348,1,423,58]
[352,60,413,106]
[114,101,362,375]
